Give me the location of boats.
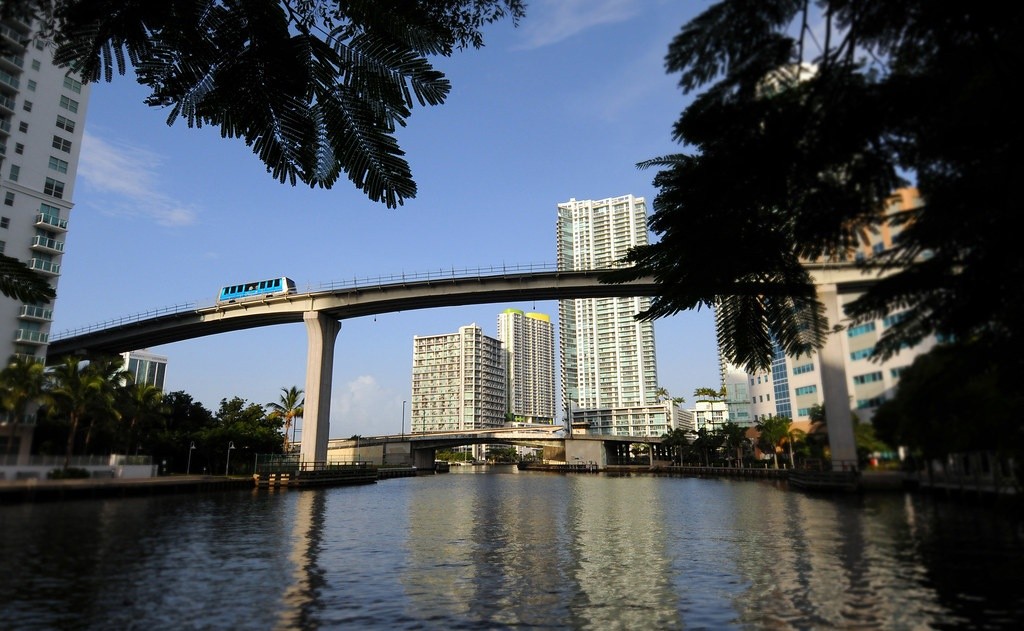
[435,461,450,473]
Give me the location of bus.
[216,277,297,307]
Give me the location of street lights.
[225,441,236,477]
[569,392,573,439]
[401,400,407,438]
[186,440,196,475]
